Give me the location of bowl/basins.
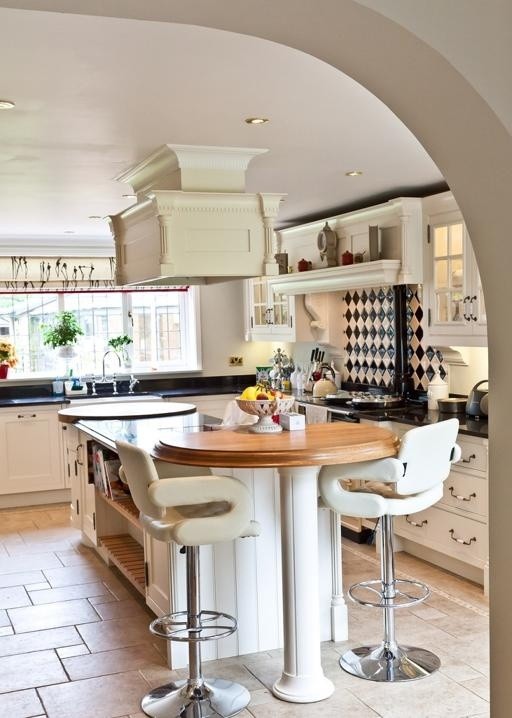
[435,397,467,414]
[441,411,467,425]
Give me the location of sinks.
[70,395,163,404]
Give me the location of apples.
[256,393,269,400]
[276,391,283,399]
[270,390,275,395]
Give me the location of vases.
[0,364,9,379]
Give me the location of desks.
[151,423,402,704]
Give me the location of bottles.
[354,252,364,264]
[272,363,308,393]
[288,258,313,274]
[341,249,352,265]
[427,408,442,424]
[325,359,342,389]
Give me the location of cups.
[274,253,288,275]
[266,263,279,276]
[426,384,450,410]
[52,381,63,396]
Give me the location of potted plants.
[40,310,85,359]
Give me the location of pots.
[322,390,405,409]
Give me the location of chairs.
[318,418,462,682]
[114,440,262,718]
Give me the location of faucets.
[127,374,140,394]
[102,350,122,382]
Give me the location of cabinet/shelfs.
[243,275,318,343]
[360,419,489,595]
[421,189,487,347]
[0,404,69,509]
[268,197,424,295]
[165,394,234,419]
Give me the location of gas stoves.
[294,398,413,417]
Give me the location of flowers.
[0,340,18,369]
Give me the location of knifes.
[310,346,326,363]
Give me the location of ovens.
[291,402,357,424]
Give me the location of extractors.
[102,143,288,288]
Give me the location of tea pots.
[309,364,337,399]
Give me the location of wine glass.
[451,271,465,321]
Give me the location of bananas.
[240,382,274,400]
